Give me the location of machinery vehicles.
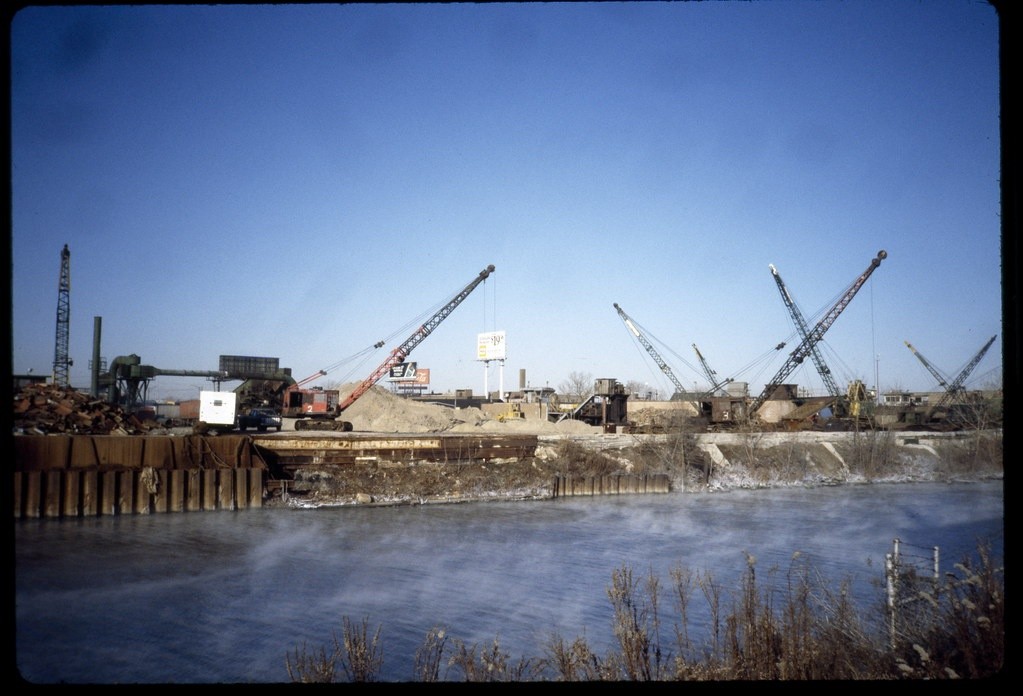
[611,245,998,434]
[517,386,571,424]
[280,264,495,432]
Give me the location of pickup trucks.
[239,410,283,432]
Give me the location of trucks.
[199,390,239,430]
